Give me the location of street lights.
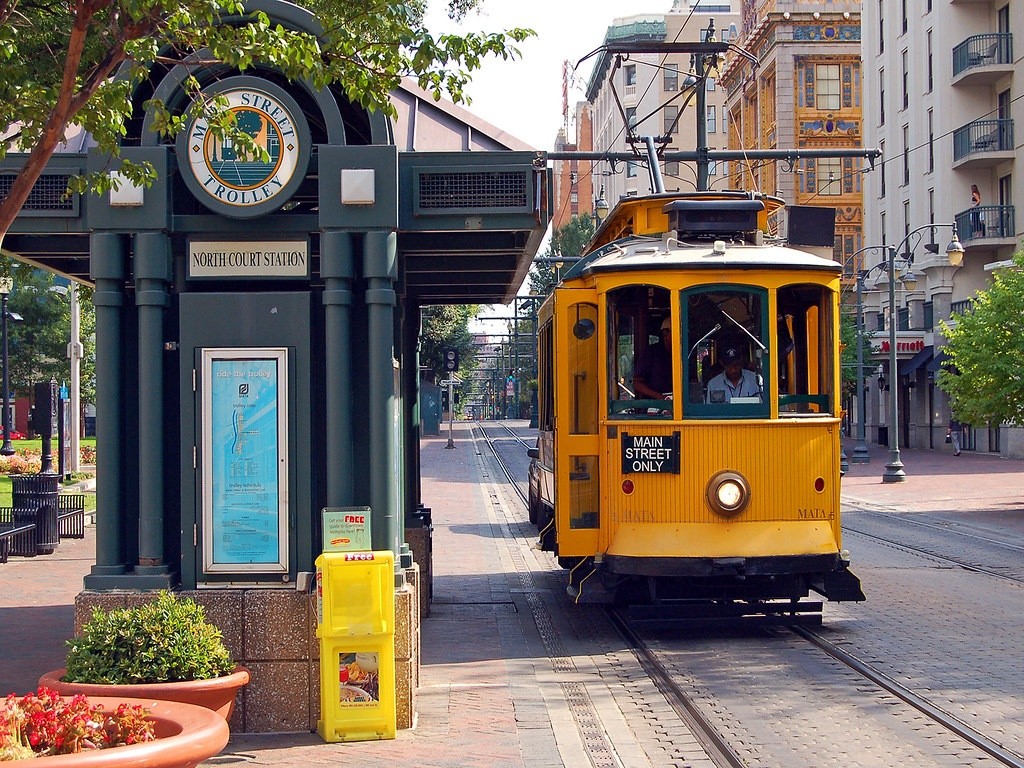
[882,222,965,484]
[491,366,497,419]
[506,319,516,418]
[0,270,24,456]
[841,244,918,464]
[529,284,539,430]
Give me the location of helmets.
[661,316,671,330]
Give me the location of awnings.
[926,345,957,372]
[900,346,933,375]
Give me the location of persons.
[947,408,967,456]
[970,185,982,238]
[702,343,763,404]
[633,317,695,400]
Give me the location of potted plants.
[38,590,249,728]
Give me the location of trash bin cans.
[313,549,398,743]
[8,474,60,554]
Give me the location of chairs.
[974,125,1003,152]
[987,212,1010,237]
[977,40,997,65]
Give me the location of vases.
[0,696,230,768]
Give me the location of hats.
[720,343,742,363]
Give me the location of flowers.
[0,686,156,761]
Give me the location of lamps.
[878,375,889,394]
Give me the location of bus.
[527,42,862,630]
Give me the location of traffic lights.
[444,347,459,372]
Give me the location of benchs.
[57,493,84,543]
[0,505,38,564]
